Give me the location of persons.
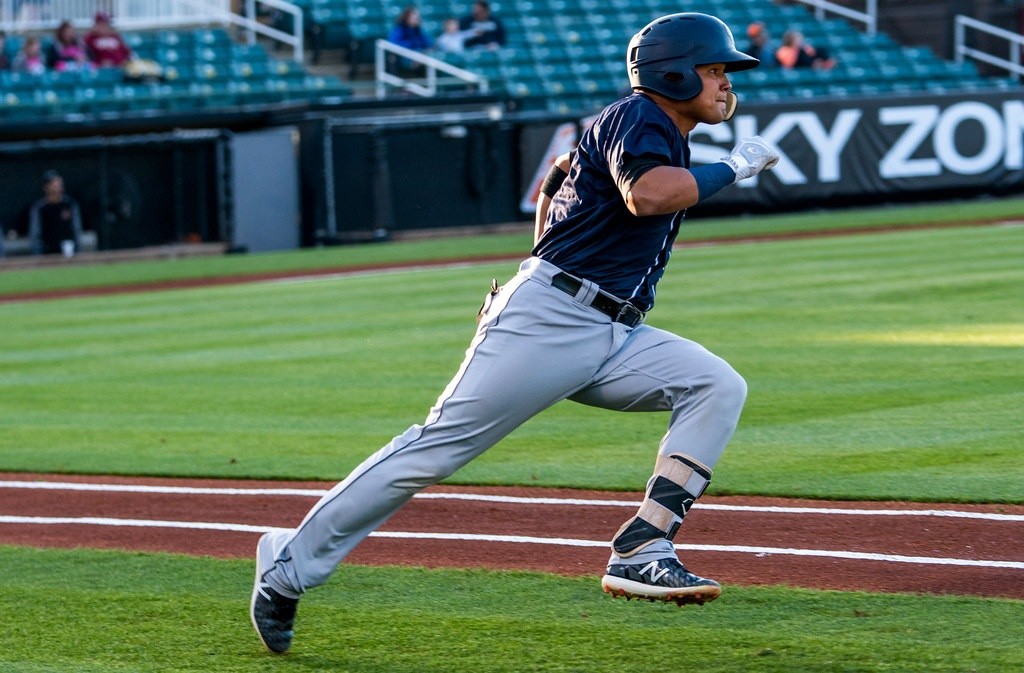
[0,8,132,74]
[389,1,506,78]
[247,9,781,658]
[745,21,836,69]
[27,168,88,257]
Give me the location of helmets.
[627,12,762,121]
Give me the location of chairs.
[2,1,1023,132]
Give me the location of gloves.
[722,135,780,184]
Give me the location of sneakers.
[599,553,723,608]
[250,530,300,654]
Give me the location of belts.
[551,272,641,330]
